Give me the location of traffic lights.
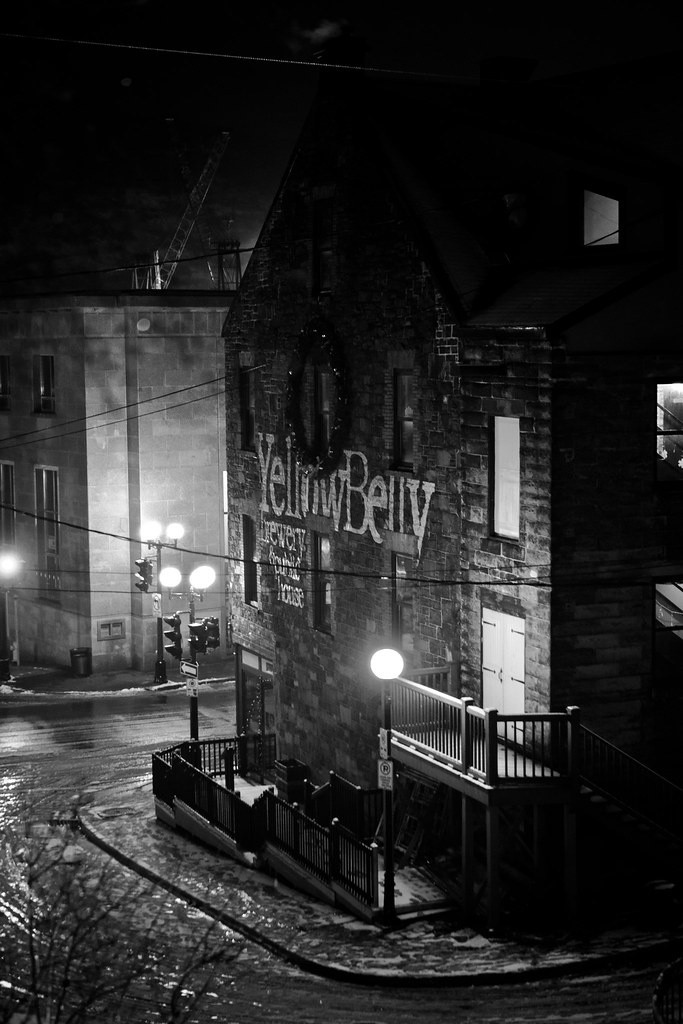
[134,559,148,592]
[207,617,219,649]
[188,622,208,656]
[162,616,182,656]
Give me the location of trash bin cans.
[67,646,92,678]
[648,879,677,937]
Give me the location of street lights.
[369,647,404,926]
[142,519,184,684]
[157,564,216,770]
